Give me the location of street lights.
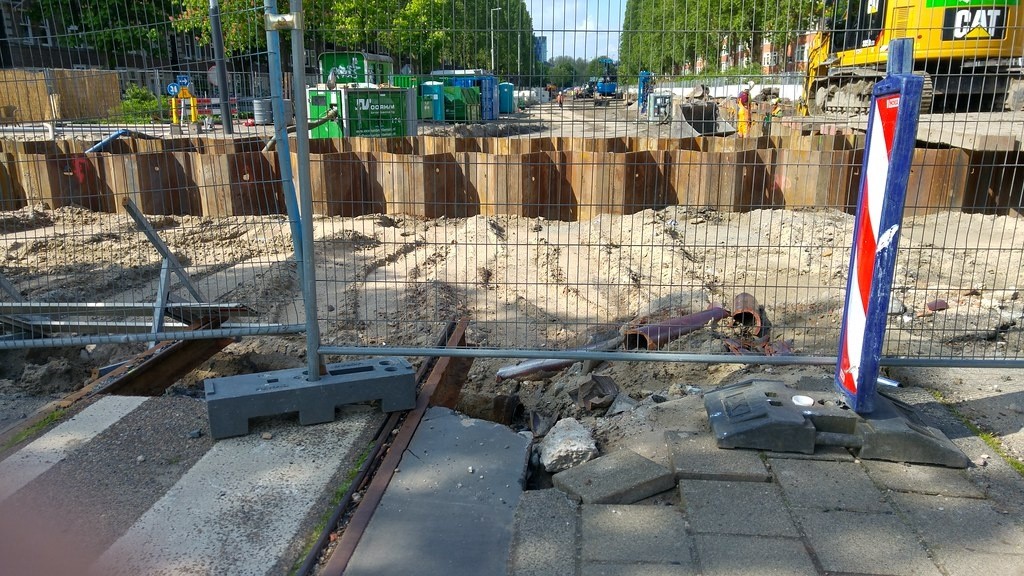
[490,8,502,74]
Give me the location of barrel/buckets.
[284,99,293,125]
[254,99,272,125]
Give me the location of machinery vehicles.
[588,59,623,96]
[796,0,1024,116]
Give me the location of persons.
[557,91,564,107]
[736,81,754,139]
[770,99,783,117]
